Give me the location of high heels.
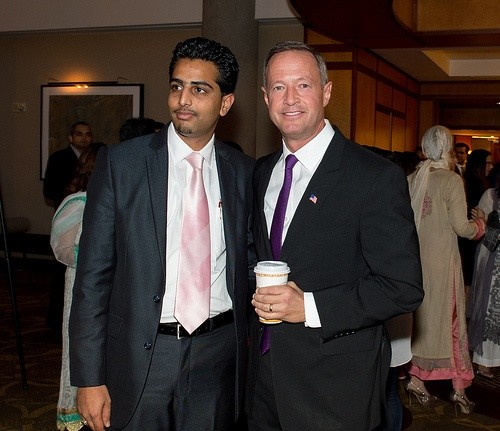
[450,390,476,419]
[406,381,438,406]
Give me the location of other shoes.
[476,370,493,378]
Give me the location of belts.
[157,309,235,340]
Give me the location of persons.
[407,124,486,418]
[414,143,500,390]
[42,117,166,350]
[68,36,255,430]
[251,41,425,431]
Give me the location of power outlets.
[12,103,27,112]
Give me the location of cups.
[253,260,291,324]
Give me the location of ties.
[173,150,210,334]
[259,154,299,357]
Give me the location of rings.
[80,420,90,426]
[268,304,275,313]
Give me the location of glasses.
[487,161,492,164]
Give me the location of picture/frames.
[40,81,145,181]
